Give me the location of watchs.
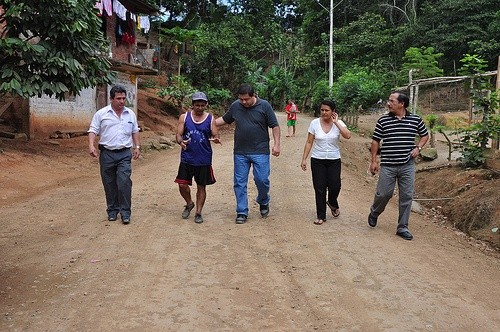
[417,146,421,150]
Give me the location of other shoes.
[236,214,246,223]
[182,202,195,219]
[109,214,117,220]
[260,204,269,216]
[195,214,203,223]
[122,216,129,223]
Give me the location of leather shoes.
[368,213,377,227]
[398,231,412,240]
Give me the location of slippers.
[314,217,326,224]
[327,201,340,217]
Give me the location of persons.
[286,98,298,137]
[301,100,351,225]
[215,84,281,224]
[368,91,429,240]
[87,85,140,223]
[174,91,221,223]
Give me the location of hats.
[192,92,207,101]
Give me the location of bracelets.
[136,146,140,149]
[178,140,182,144]
